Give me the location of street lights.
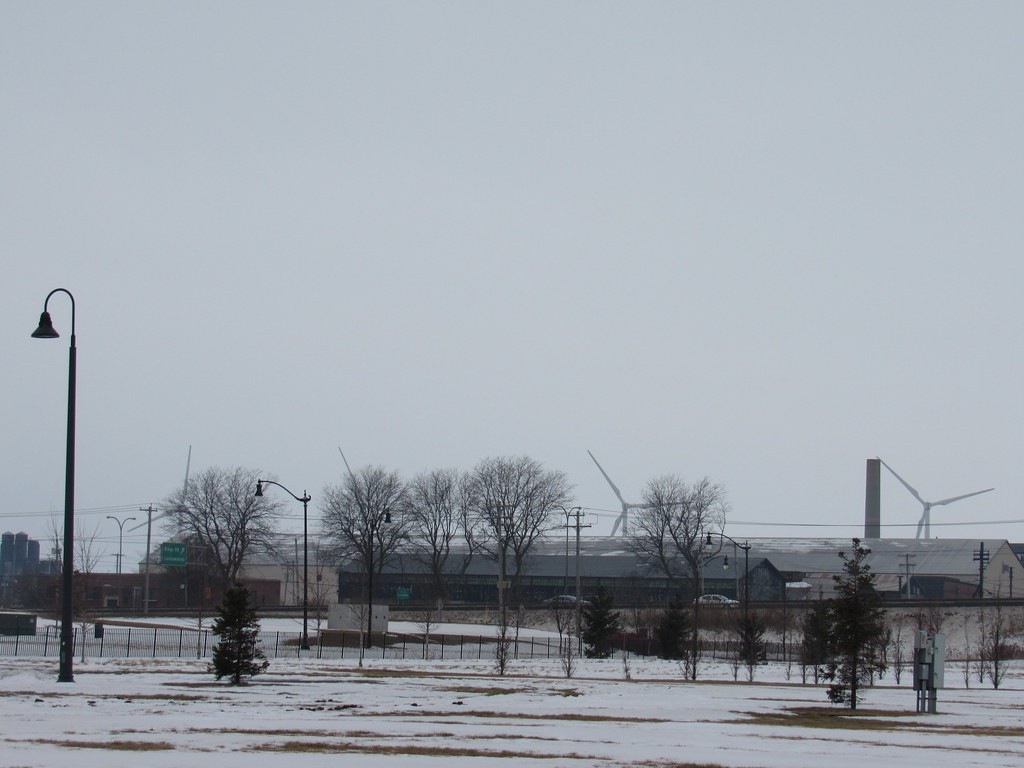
[31,289,77,685]
[704,529,751,679]
[551,503,584,669]
[107,514,136,608]
[341,510,393,637]
[254,477,310,651]
